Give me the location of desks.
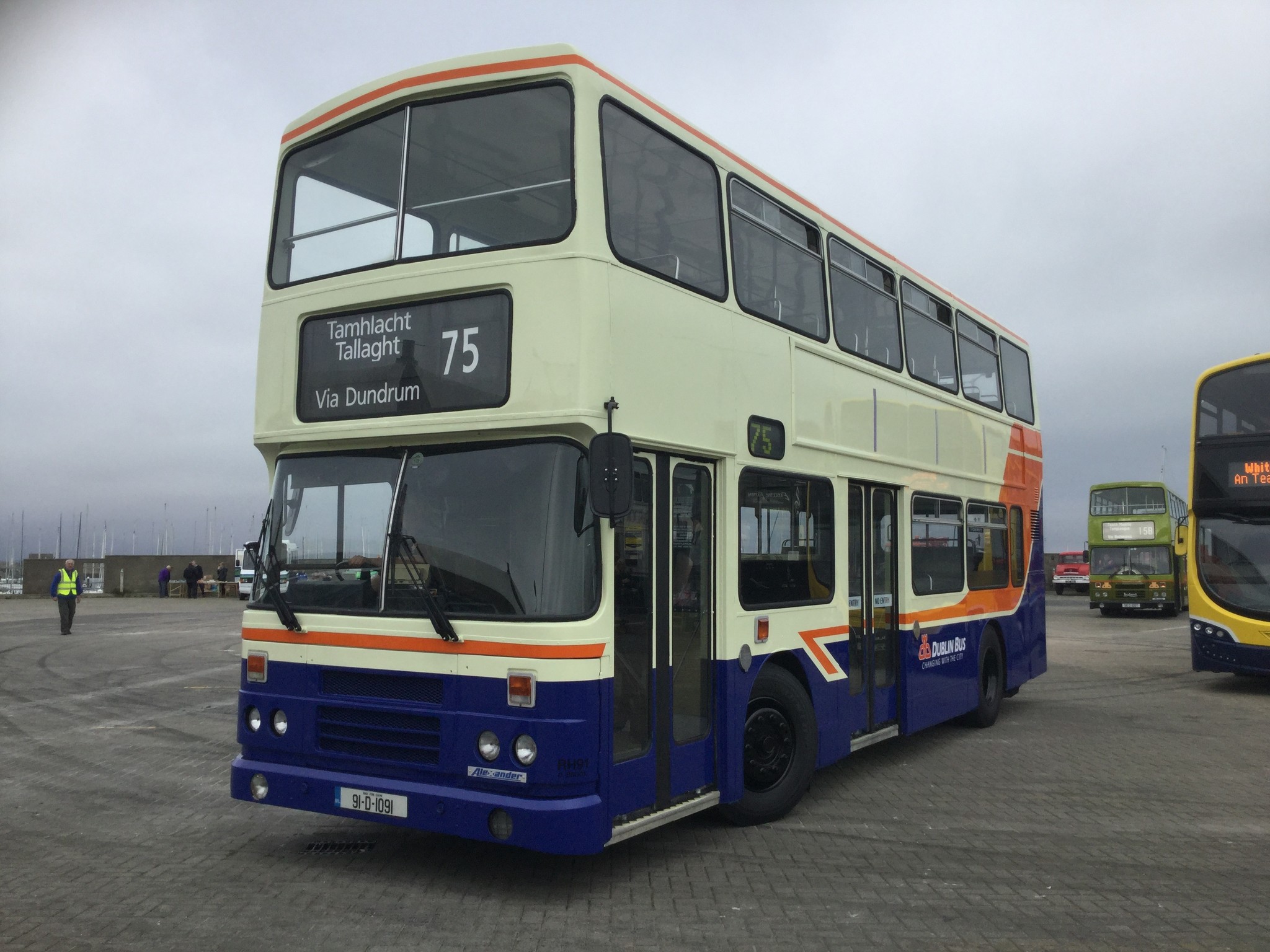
[169,580,238,598]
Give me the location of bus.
[1173,351,1270,679]
[1082,480,1189,617]
[234,539,299,601]
[228,42,1048,858]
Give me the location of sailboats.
[0,502,381,595]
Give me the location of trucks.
[1051,551,1090,595]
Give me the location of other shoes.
[61,631,67,634]
[67,631,71,634]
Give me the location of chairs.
[632,253,680,279]
[912,574,932,595]
[781,537,816,553]
[692,278,1016,415]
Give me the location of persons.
[51,559,83,635]
[349,500,433,568]
[183,561,206,598]
[1097,554,1114,569]
[217,562,227,598]
[158,565,174,597]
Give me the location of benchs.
[907,538,976,560]
